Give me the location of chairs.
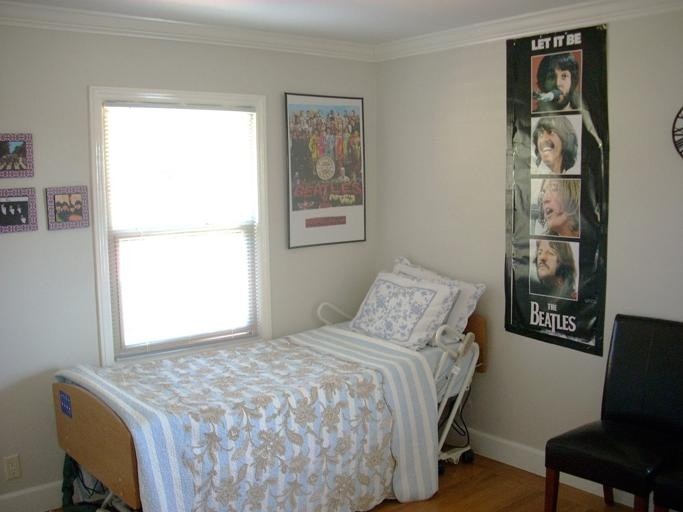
[545,314,682,511]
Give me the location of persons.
[531,117,577,174]
[538,179,579,237]
[532,241,577,299]
[8,204,20,224]
[287,110,361,184]
[0,203,8,225]
[61,202,70,221]
[69,206,74,213]
[16,202,26,224]
[531,54,579,111]
[54,201,63,221]
[69,201,82,221]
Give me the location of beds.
[53,313,488,511]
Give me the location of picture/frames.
[285,93,365,249]
[0,133,39,232]
[46,186,90,231]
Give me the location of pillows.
[349,258,486,353]
